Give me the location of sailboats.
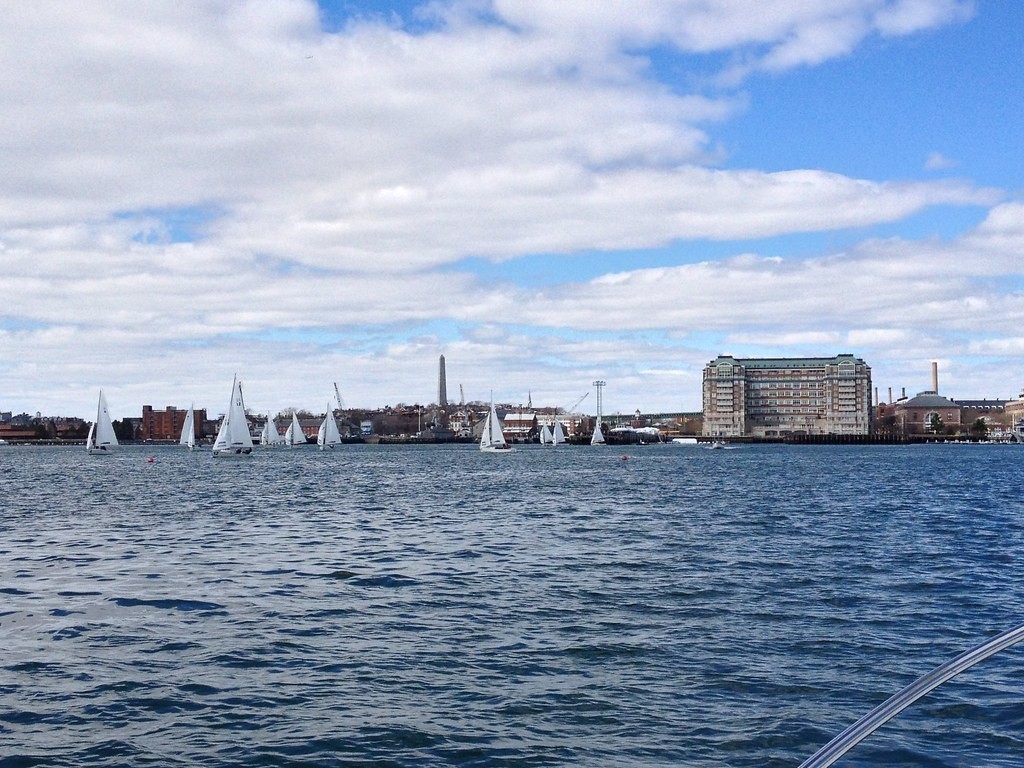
[259,410,281,449]
[212,373,254,457]
[316,403,342,451]
[552,418,569,446]
[480,390,516,453]
[539,421,553,446]
[283,411,307,449]
[180,402,203,452]
[590,420,607,446]
[85,387,119,455]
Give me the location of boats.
[712,443,724,449]
